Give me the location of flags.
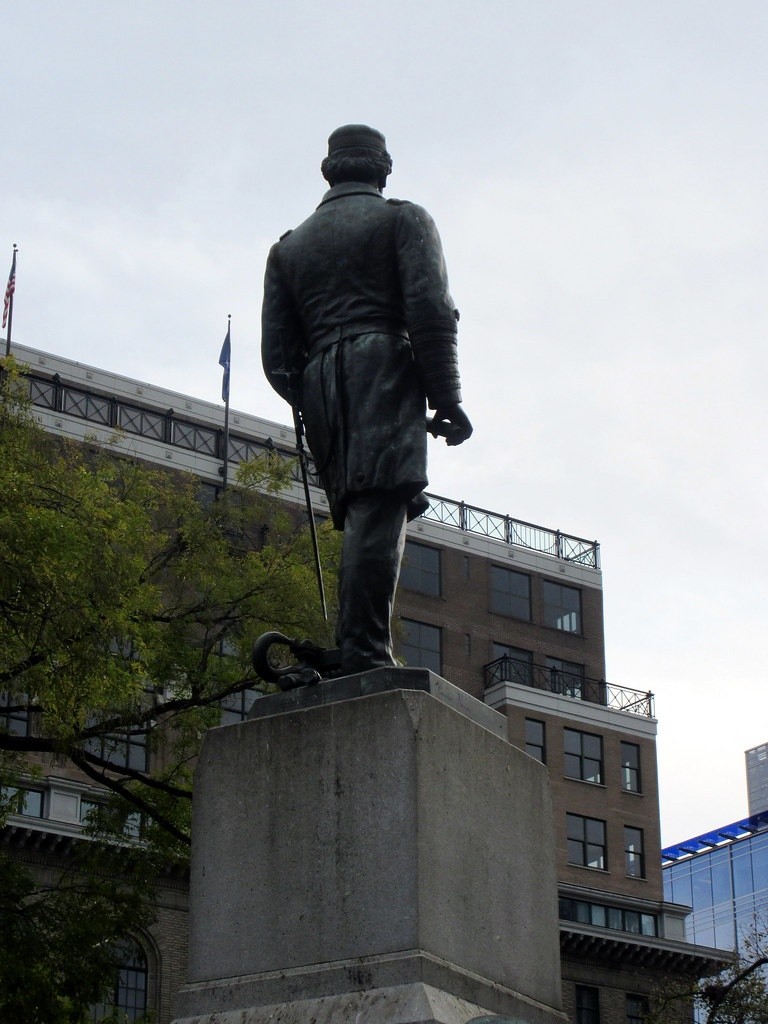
[2,254,15,328]
[218,332,232,402]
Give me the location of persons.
[261,124,474,669]
